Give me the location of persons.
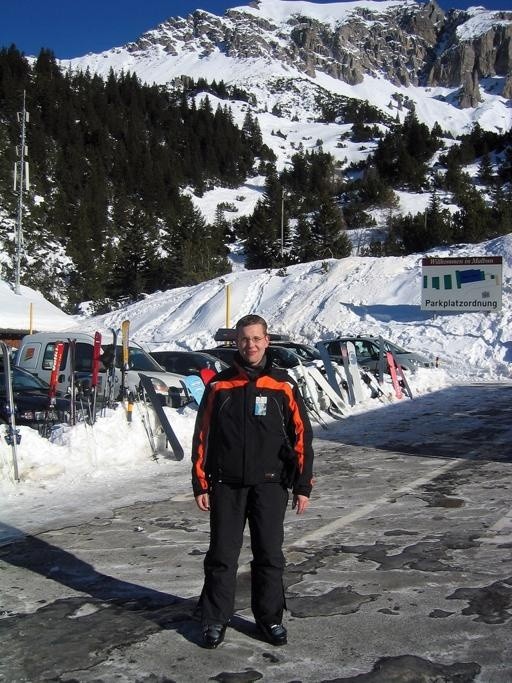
[191,315,315,650]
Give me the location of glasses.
[236,334,266,343]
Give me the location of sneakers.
[254,617,288,646]
[200,620,227,650]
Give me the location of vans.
[15,329,195,408]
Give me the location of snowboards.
[200,368,217,386]
[317,342,344,401]
[378,334,384,386]
[308,367,353,417]
[385,351,403,399]
[296,356,321,417]
[339,341,365,406]
[138,372,184,460]
[215,360,223,373]
[389,343,412,399]
[184,374,206,406]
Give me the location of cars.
[0,363,91,426]
[193,328,434,387]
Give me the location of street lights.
[281,189,291,257]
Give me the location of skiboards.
[40,340,65,439]
[122,320,130,404]
[108,327,121,409]
[87,331,102,426]
[67,337,77,426]
[0,342,22,480]
[301,396,329,431]
